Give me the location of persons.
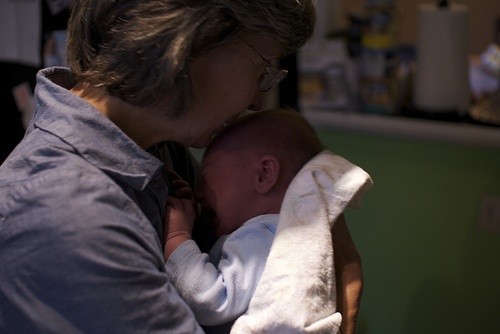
[0,0,365,334]
[163,108,346,334]
